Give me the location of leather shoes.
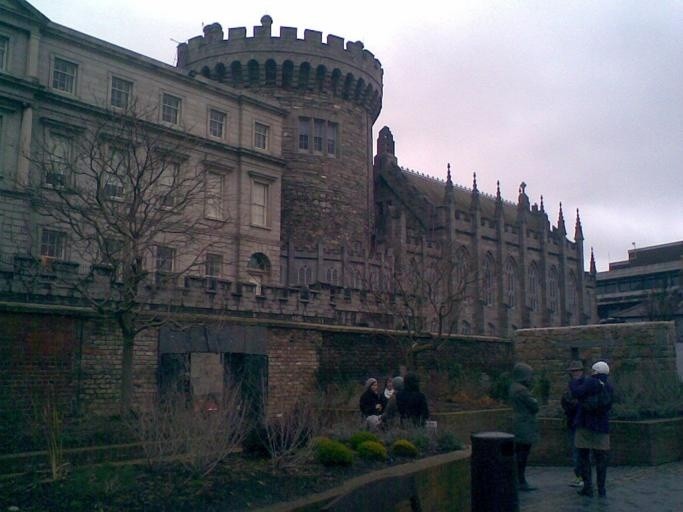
[519,483,537,491]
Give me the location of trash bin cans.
[470,432,520,512]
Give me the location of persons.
[569,361,613,497]
[561,361,590,486]
[360,373,429,429]
[509,363,540,490]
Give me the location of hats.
[365,377,376,388]
[564,360,586,372]
[391,377,405,390]
[592,362,609,375]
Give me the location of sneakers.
[577,487,594,497]
[568,475,584,486]
[596,488,606,495]
